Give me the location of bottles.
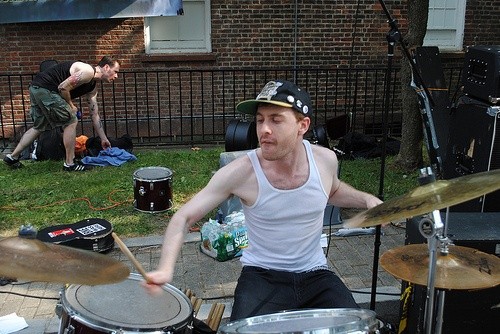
[199,210,247,262]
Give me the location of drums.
[54,271,195,334]
[132,166,175,215]
[218,306,385,334]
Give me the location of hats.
[234,80,311,115]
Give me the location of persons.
[4,56,121,171]
[143,78,390,322]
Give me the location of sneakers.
[3,154,24,168]
[63,162,93,171]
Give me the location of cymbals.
[341,167,500,230]
[379,242,500,291]
[0,235,131,286]
